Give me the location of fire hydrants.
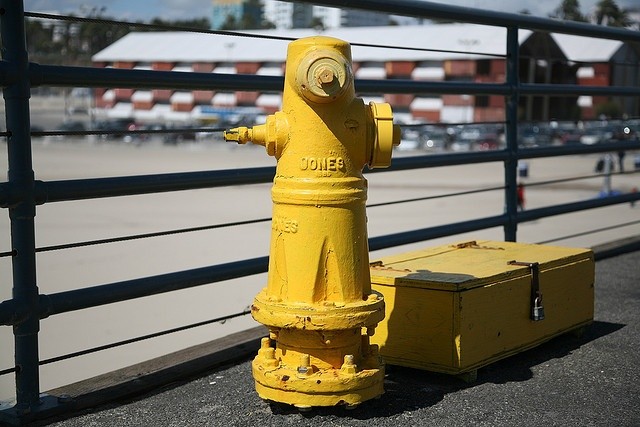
[223,35,400,408]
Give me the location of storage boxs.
[367,237,597,386]
